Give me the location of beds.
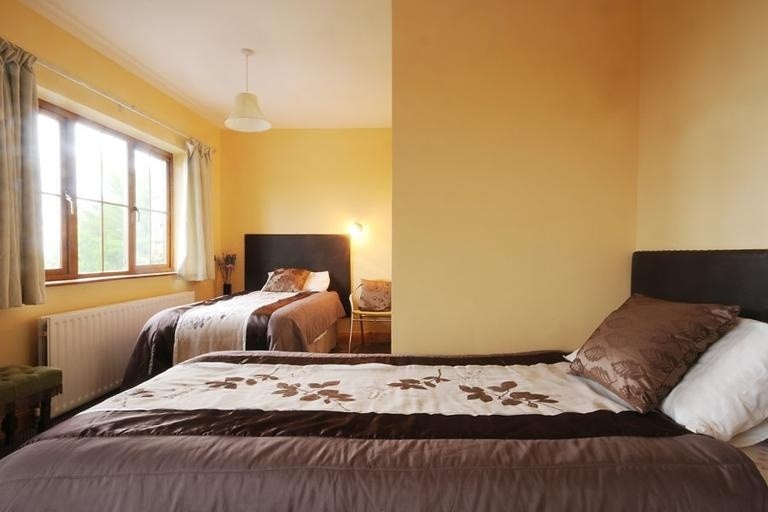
[0,250,761,511]
[122,234,351,389]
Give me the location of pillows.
[260,266,306,292]
[359,278,392,313]
[570,294,739,416]
[303,271,331,290]
[658,313,768,450]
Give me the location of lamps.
[351,223,362,232]
[223,49,272,134]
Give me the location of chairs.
[347,282,391,354]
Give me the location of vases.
[223,283,231,295]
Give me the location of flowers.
[214,253,236,284]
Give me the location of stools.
[0,363,63,446]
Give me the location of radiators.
[34,292,194,419]
